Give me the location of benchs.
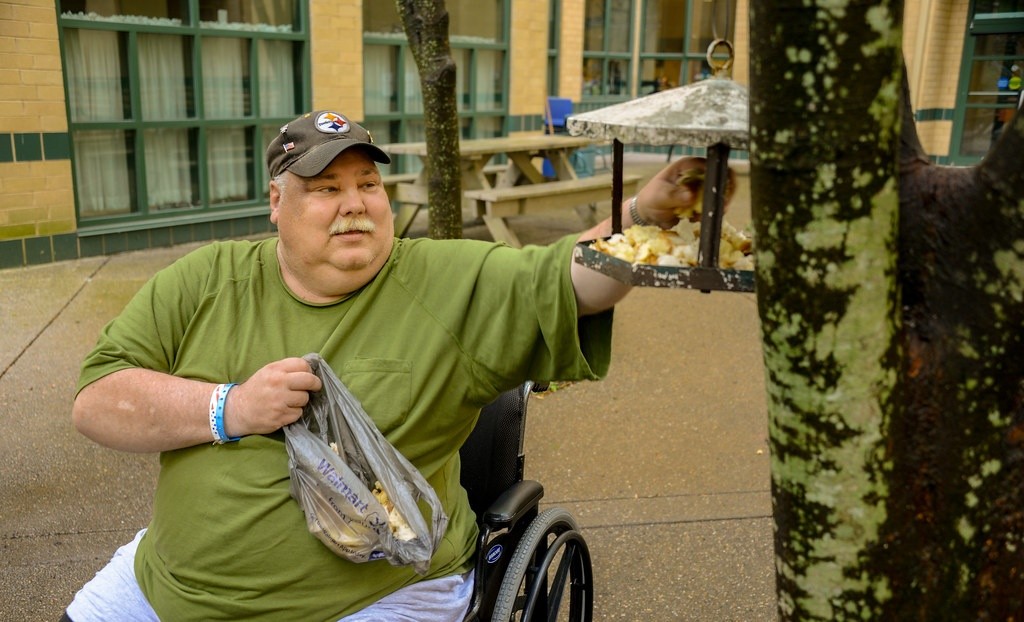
[464,172,639,218]
[380,162,510,198]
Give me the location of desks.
[380,132,640,248]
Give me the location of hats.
[266,109,391,179]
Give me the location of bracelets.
[209,384,239,444]
[630,195,646,226]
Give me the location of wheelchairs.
[459,378,593,622]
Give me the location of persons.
[60,110,738,619]
